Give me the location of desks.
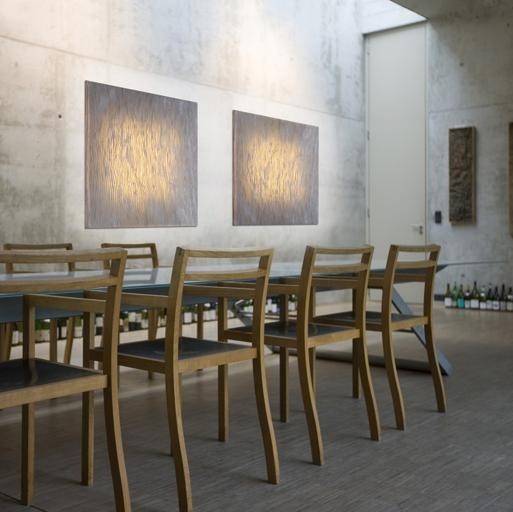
[0,260,456,377]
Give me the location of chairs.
[82,246,279,512]
[307,243,442,431]
[0,248,133,511]
[101,243,159,270]
[4,243,76,275]
[217,244,382,467]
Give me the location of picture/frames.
[83,80,199,230]
[232,110,319,226]
[449,125,476,223]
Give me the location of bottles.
[493,286,500,310]
[452,281,457,307]
[500,284,507,311]
[507,287,513,311]
[444,283,452,308]
[464,289,470,309]
[470,281,479,309]
[457,283,464,308]
[479,288,486,310]
[486,287,493,310]
